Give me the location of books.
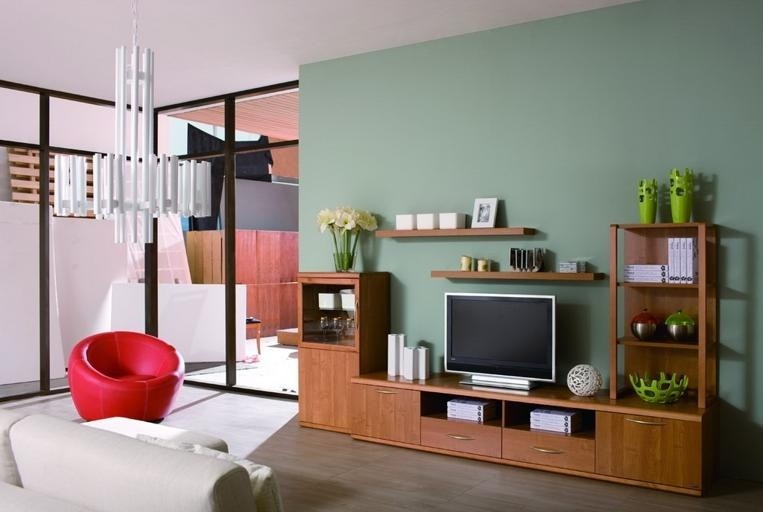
[668,238,693,284]
[623,265,668,283]
[530,407,583,434]
[447,398,496,423]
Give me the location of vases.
[332,252,357,273]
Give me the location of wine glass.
[331,317,344,342]
[347,318,355,329]
[321,317,329,340]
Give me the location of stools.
[246,317,262,355]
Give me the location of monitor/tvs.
[445,294,559,391]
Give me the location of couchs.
[1,409,275,512]
[69,331,185,421]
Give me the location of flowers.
[317,208,378,270]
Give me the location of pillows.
[134,432,286,512]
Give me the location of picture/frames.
[471,198,498,228]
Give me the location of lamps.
[53,1,212,246]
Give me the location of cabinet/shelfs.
[344,373,718,498]
[295,270,392,436]
[610,221,720,408]
